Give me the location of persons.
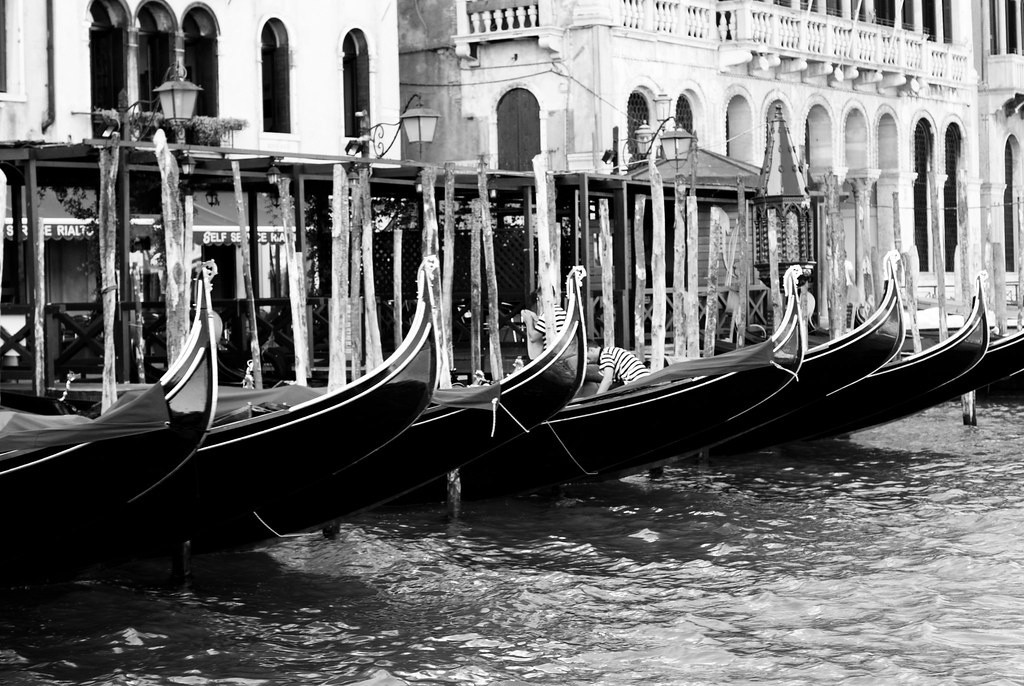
[844,251,855,287]
[522,285,567,352]
[586,339,651,394]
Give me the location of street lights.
[601,114,697,344]
[117,58,203,386]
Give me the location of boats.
[0,260,218,570]
[118,229,1024,557]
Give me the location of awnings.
[3,183,327,247]
